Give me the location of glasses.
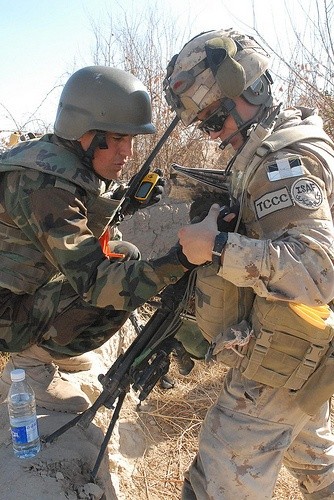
[197,105,229,134]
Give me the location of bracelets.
[212,232,228,265]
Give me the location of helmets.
[54,66,157,141]
[170,30,271,127]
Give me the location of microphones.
[219,106,266,150]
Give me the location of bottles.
[7,368,42,460]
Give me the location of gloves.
[114,166,164,215]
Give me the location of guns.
[41,264,196,485]
[110,114,182,226]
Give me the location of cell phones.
[133,172,159,204]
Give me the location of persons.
[162,28,334,500]
[0,66,235,411]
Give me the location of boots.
[0,344,90,413]
[53,353,92,372]
